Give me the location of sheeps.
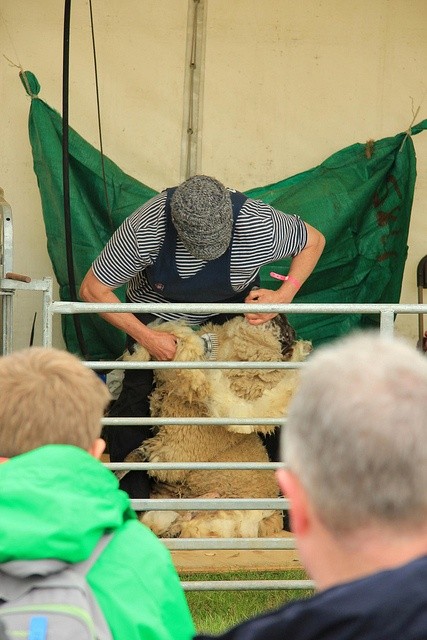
[105,285,313,540]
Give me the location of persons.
[217,330,427,640]
[0,347,197,639]
[78,175,327,528]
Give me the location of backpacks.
[2,532,117,639]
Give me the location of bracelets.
[271,273,303,290]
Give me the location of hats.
[169,172,234,261]
